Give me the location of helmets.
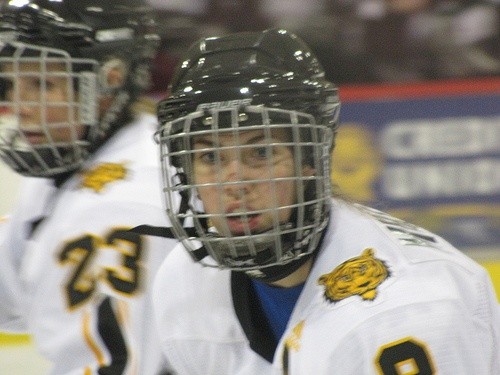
[0,0,153,60]
[153,28,341,168]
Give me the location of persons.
[0,0,211,374]
[143,27,500,374]
